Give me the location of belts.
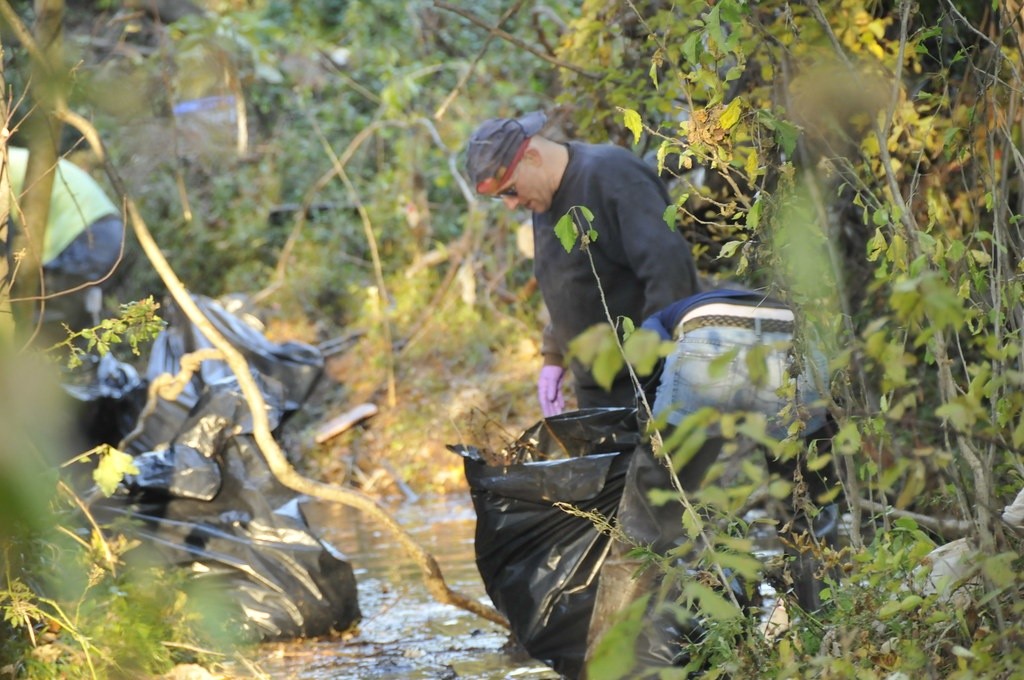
[673,315,797,341]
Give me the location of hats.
[465,112,546,193]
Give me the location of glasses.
[492,159,521,198]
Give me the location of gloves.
[538,366,565,418]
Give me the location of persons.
[0,146,125,322]
[465,109,699,416]
[581,288,841,680]
